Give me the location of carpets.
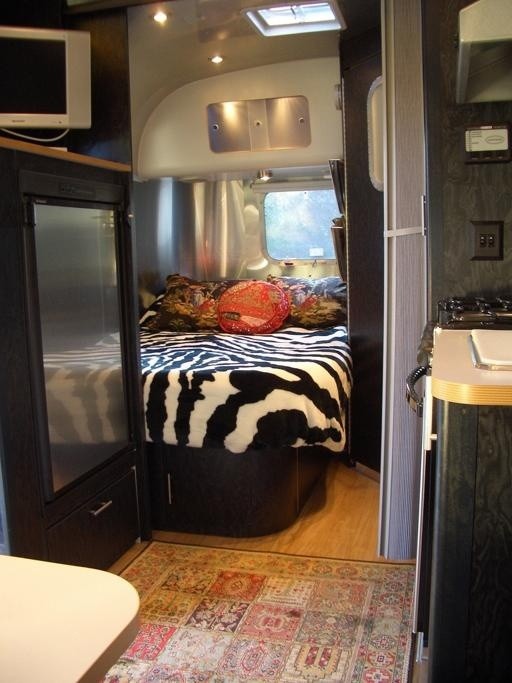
[99,539,416,683]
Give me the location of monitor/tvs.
[0,27,93,132]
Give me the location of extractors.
[454,0,512,106]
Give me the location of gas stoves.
[436,294,512,328]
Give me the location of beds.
[139,281,354,537]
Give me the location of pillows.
[140,273,347,336]
